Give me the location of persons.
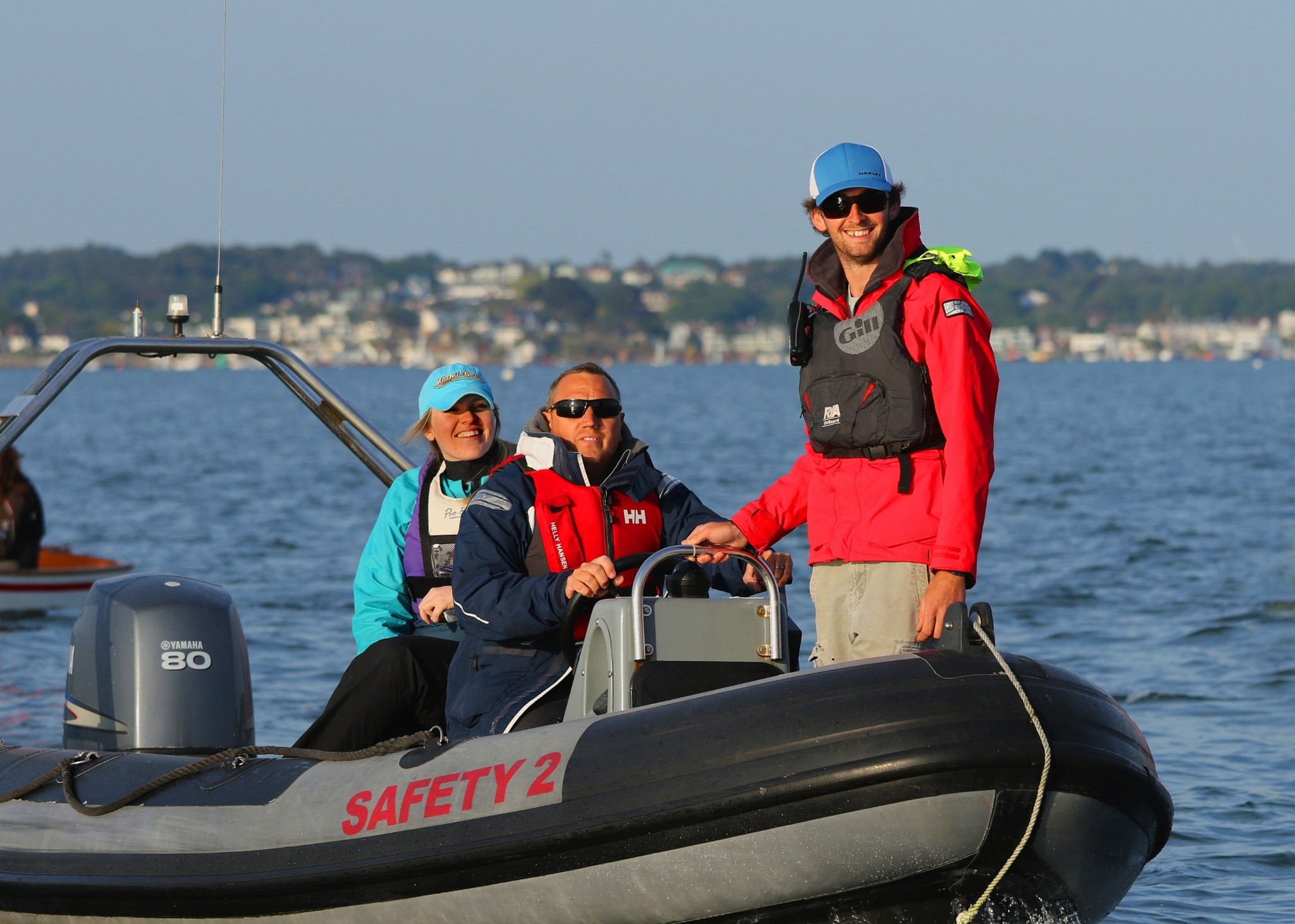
[282,364,518,758]
[680,142,1000,669]
[446,363,792,737]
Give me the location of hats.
[419,363,495,418]
[809,142,894,206]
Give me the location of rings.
[775,565,785,570]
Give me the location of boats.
[1,2,1177,924]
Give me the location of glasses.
[548,397,623,417]
[819,191,890,219]
